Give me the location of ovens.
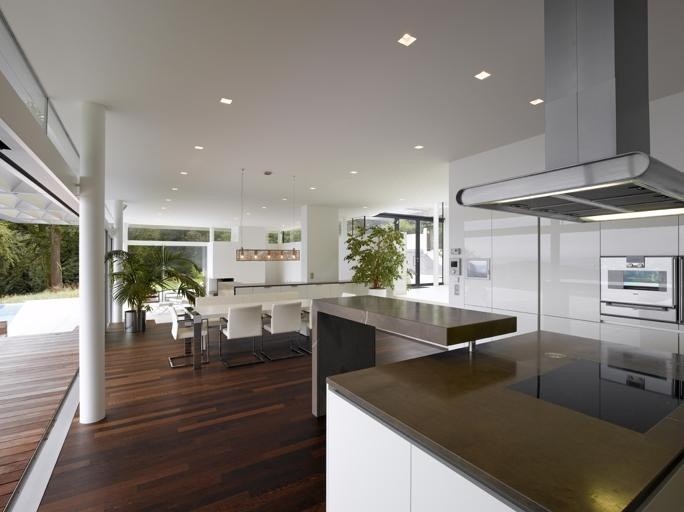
[599,340,684,400]
[599,255,684,324]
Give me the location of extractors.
[452,0,684,223]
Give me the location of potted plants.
[342,223,408,298]
[104,244,206,333]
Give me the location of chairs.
[164,291,311,373]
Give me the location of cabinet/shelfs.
[322,383,519,512]
